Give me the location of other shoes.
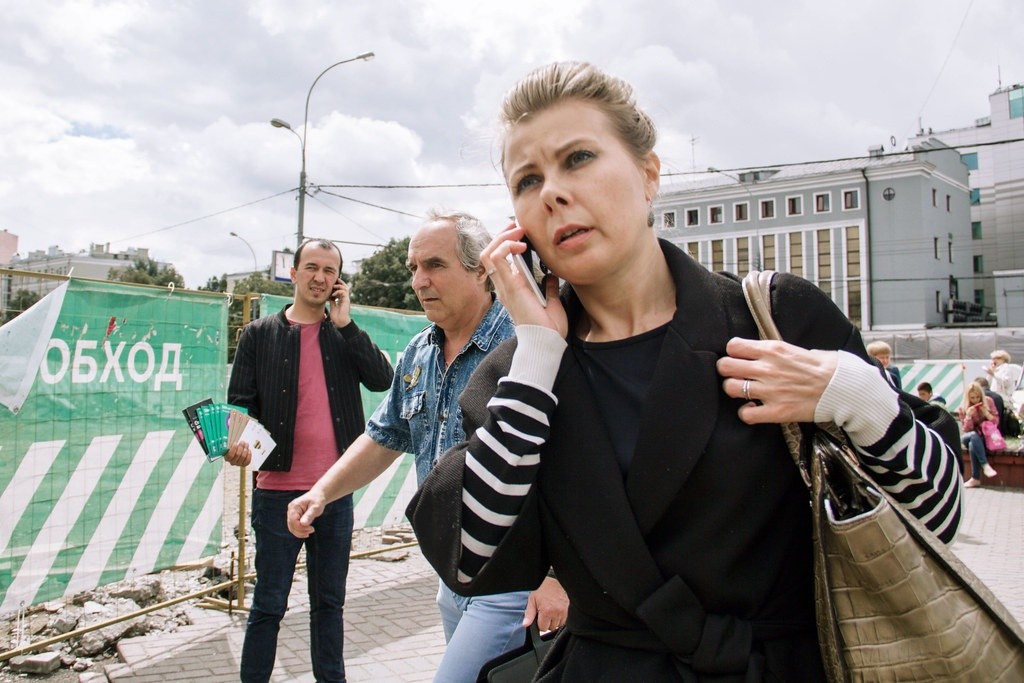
[964,477,982,488]
[982,463,997,478]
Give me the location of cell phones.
[330,275,342,301]
[973,402,983,411]
[513,236,548,309]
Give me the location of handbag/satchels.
[474,614,561,683]
[1002,406,1021,437]
[742,270,1024,683]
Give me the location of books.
[182,398,277,470]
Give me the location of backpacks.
[979,420,1007,450]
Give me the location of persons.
[287,213,571,683]
[986,349,1022,408]
[865,341,900,377]
[917,382,947,406]
[959,377,1004,488]
[227,238,394,683]
[403,61,964,683]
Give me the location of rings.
[743,380,752,400]
[486,267,497,275]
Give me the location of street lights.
[270,52,375,247]
[230,232,257,270]
